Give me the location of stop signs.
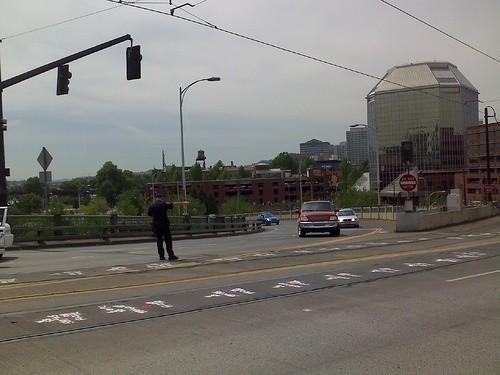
[399,174,417,191]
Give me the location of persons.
[147,193,192,261]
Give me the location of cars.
[336,208,359,228]
[298,201,340,236]
[0,206,15,263]
[256,213,280,226]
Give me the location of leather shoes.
[160,258,165,260]
[169,256,178,260]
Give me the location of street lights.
[180,77,220,215]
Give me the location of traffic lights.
[126,45,142,80]
[401,141,413,163]
[57,64,72,95]
